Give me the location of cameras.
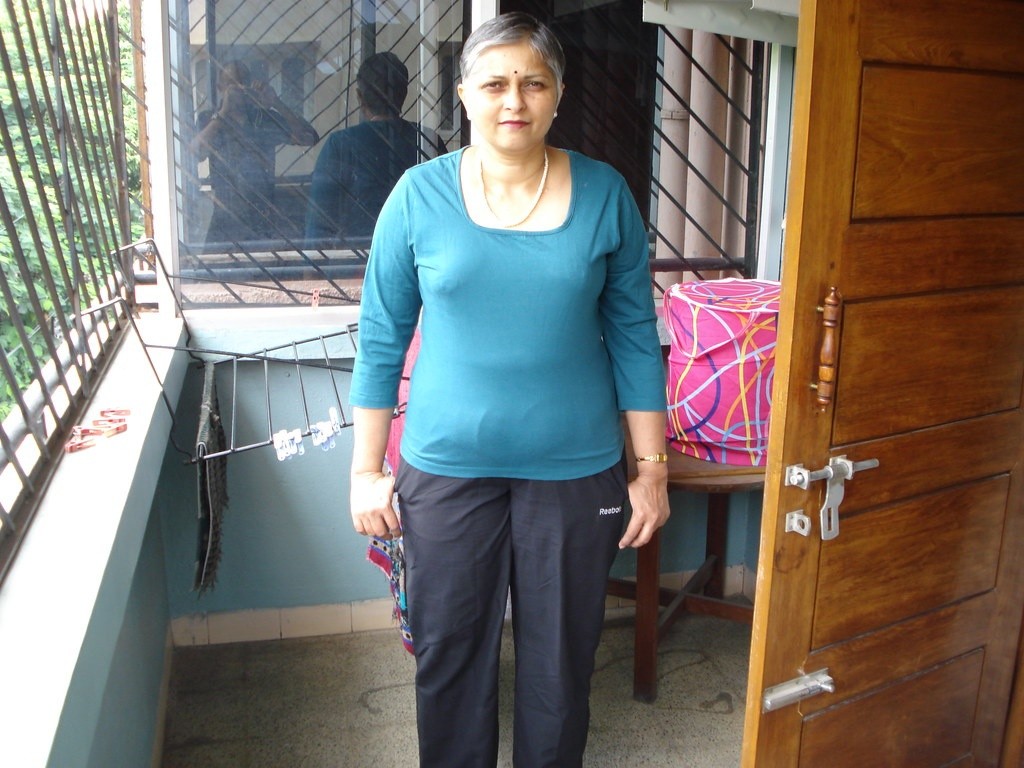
[229,86,261,109]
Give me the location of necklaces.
[369,115,380,121]
[476,149,548,226]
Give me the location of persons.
[188,61,319,284]
[302,51,448,280]
[349,12,670,768]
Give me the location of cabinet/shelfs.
[42,237,415,465]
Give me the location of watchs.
[635,453,668,463]
[211,113,222,122]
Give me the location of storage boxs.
[665,278,784,467]
